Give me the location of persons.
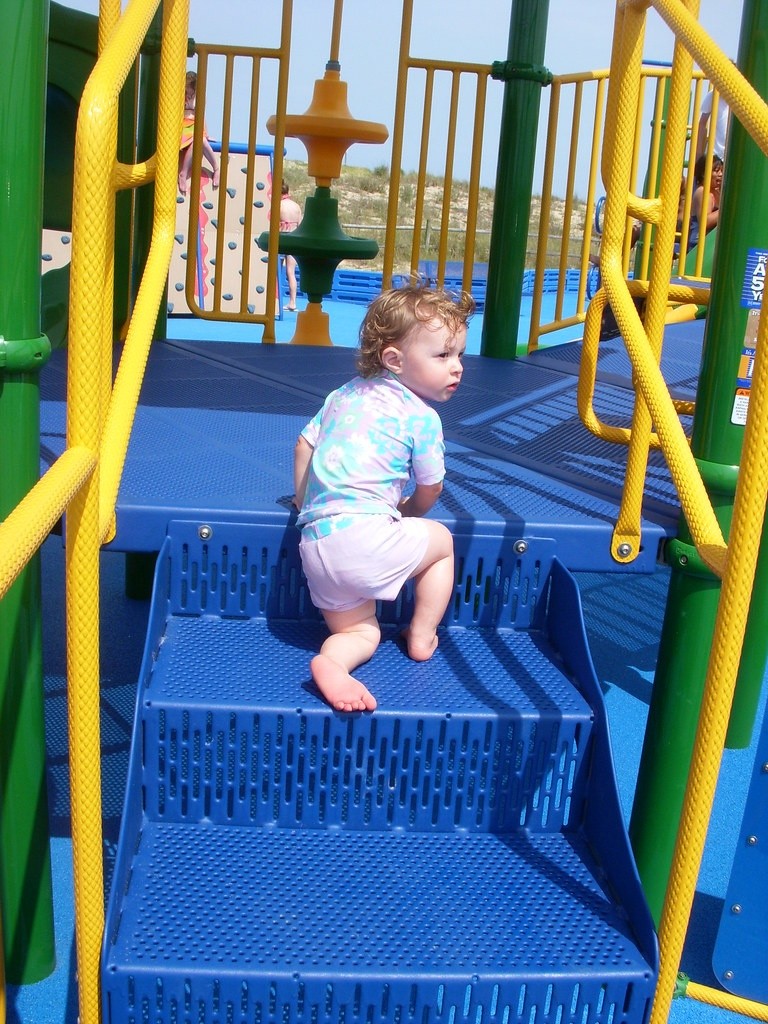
[178,71,220,192]
[291,273,477,712]
[589,154,724,266]
[275,183,301,310]
